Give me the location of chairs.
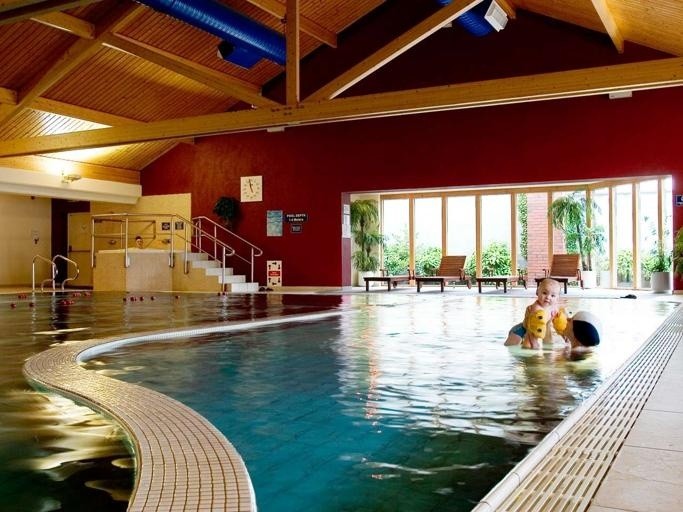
[363,253,584,295]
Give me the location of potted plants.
[348,198,375,286]
[351,230,386,286]
[645,254,672,293]
[545,195,596,289]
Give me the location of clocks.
[240,175,263,202]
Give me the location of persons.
[521,305,603,354]
[134,236,143,249]
[504,278,567,350]
